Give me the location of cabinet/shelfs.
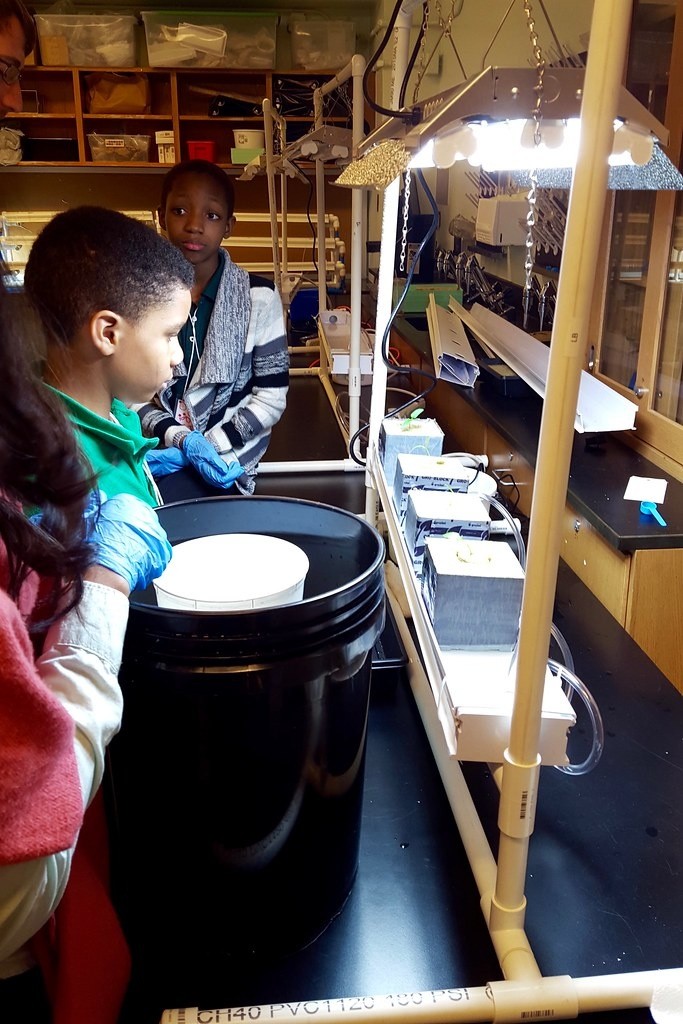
[484,425,682,695]
[0,66,349,169]
[390,329,488,453]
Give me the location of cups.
[232,129,265,149]
[187,140,216,162]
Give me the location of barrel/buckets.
[152,534,310,612]
[61,495,385,953]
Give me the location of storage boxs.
[164,145,170,163]
[230,148,265,165]
[169,145,175,163]
[20,137,78,161]
[185,140,218,163]
[393,284,463,312]
[85,132,152,162]
[156,138,175,145]
[32,14,139,67]
[155,131,174,138]
[158,145,165,164]
[140,10,281,70]
[286,19,356,71]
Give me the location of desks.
[119,331,683,1024]
[392,313,683,556]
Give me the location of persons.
[121,158,289,504]
[0,0,190,1024]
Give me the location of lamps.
[280,82,367,165]
[402,0,671,170]
[235,122,310,185]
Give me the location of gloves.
[145,446,189,478]
[87,492,173,592]
[183,429,245,489]
[28,492,106,555]
